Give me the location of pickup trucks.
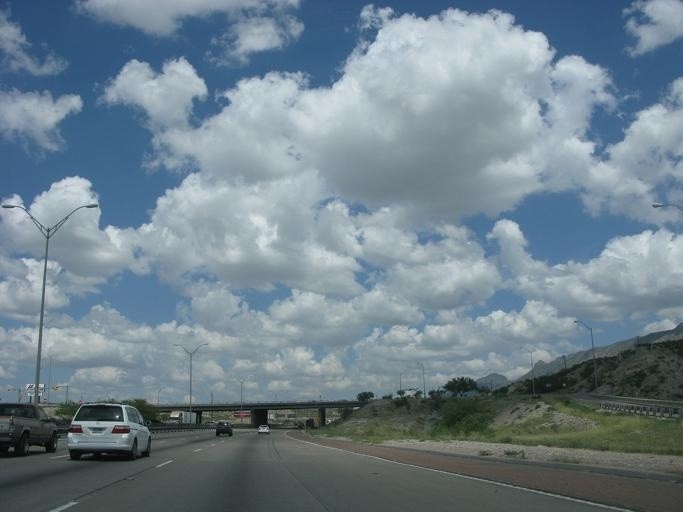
[0,402,59,458]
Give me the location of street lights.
[1,202,99,406]
[172,343,208,412]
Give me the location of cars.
[215,421,270,437]
[67,402,152,461]
